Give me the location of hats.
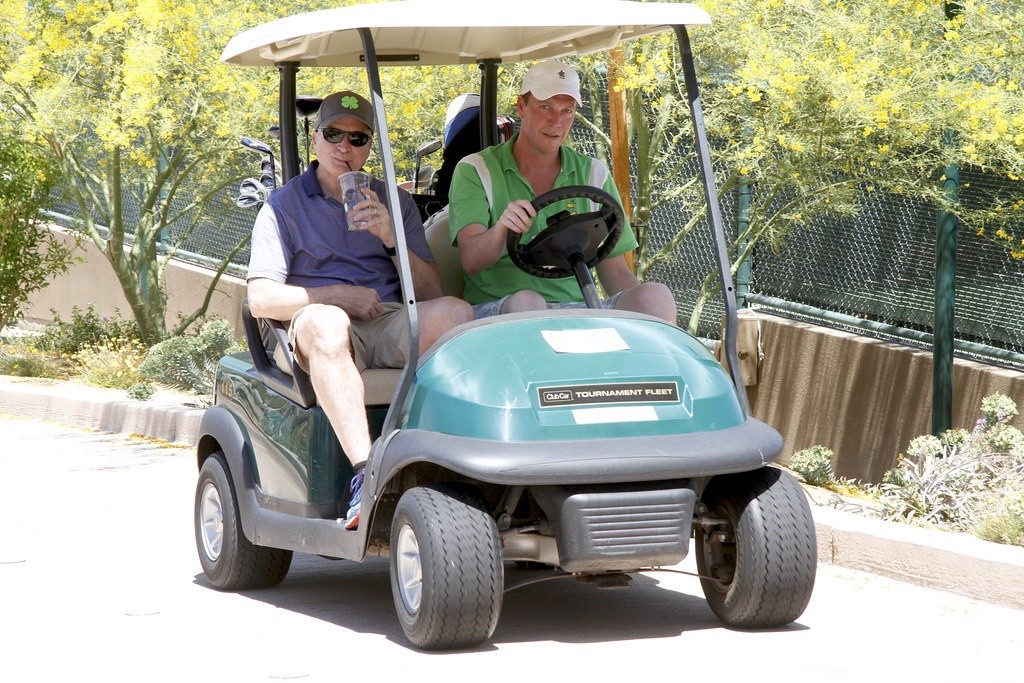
[520,61,583,107]
[314,91,374,135]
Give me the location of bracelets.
[382,243,396,256]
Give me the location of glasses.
[316,128,372,147]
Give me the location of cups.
[337,171,370,231]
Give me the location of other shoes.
[345,468,366,528]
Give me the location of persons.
[449,62,676,326]
[246,92,475,530]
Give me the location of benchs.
[241,194,465,406]
